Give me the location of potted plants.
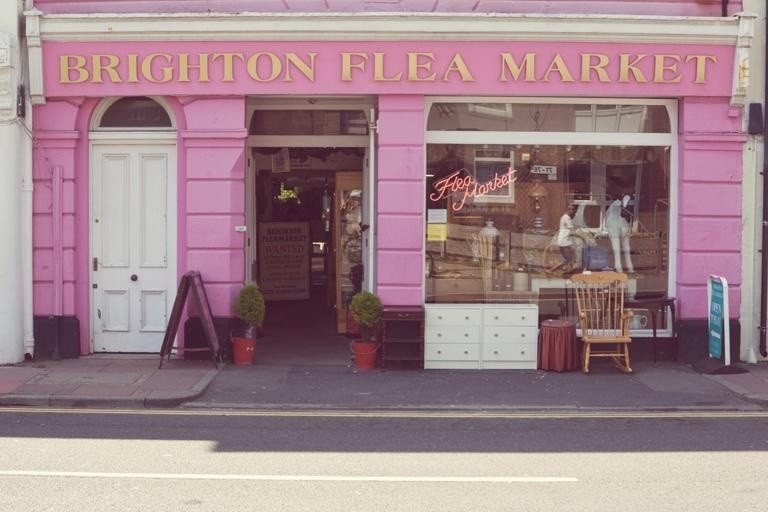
[350,293,382,369]
[231,287,267,365]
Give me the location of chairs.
[572,272,634,376]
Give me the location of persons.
[604,186,636,274]
[545,202,587,275]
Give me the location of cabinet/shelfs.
[382,304,539,368]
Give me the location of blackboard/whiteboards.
[189,271,220,355]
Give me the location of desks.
[620,297,677,364]
